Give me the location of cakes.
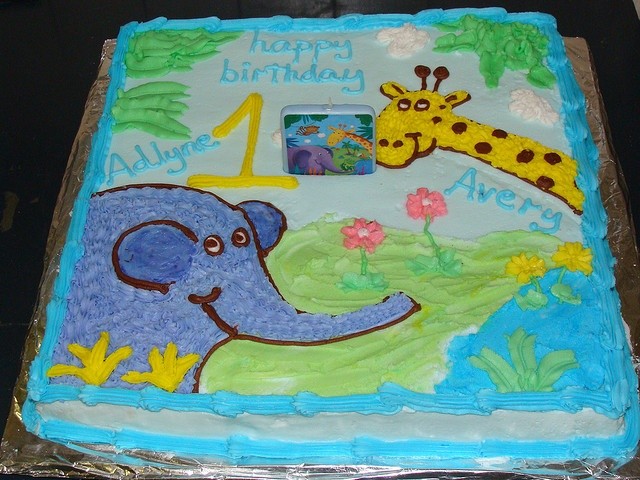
[20,6,636,480]
